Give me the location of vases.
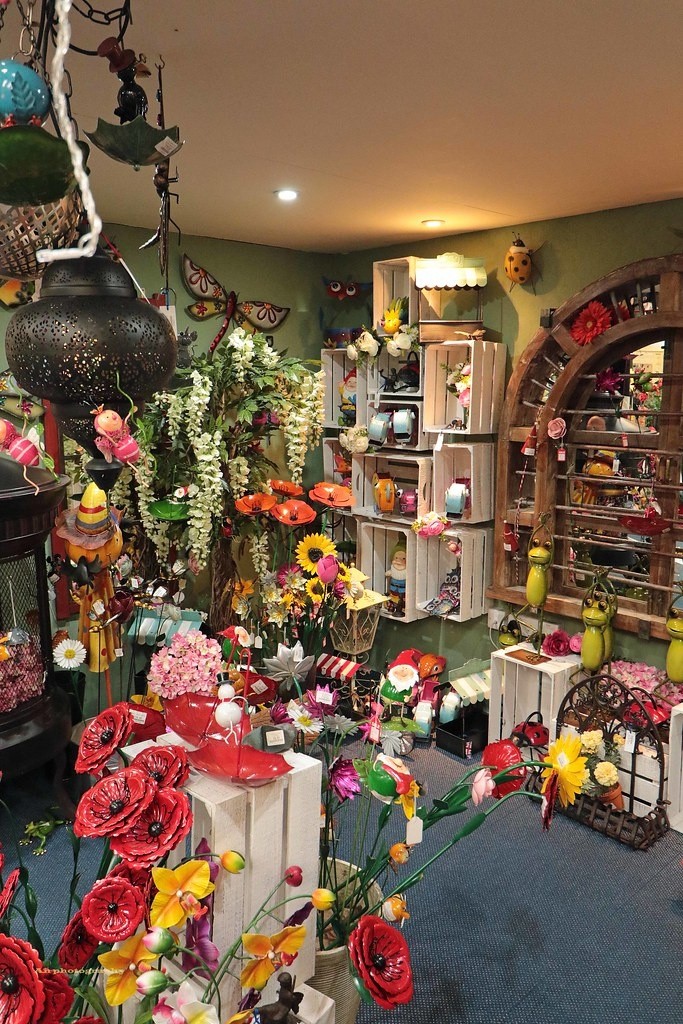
[306,851,395,1023]
[601,783,624,810]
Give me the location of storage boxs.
[360,522,431,623]
[322,437,355,515]
[321,509,369,570]
[488,641,592,772]
[416,527,493,622]
[432,442,495,525]
[364,401,463,453]
[351,452,434,526]
[372,254,488,342]
[319,346,369,433]
[423,337,510,436]
[368,342,425,398]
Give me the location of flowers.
[1,303,683,1023]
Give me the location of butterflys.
[180,251,291,353]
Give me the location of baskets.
[0,188,85,282]
